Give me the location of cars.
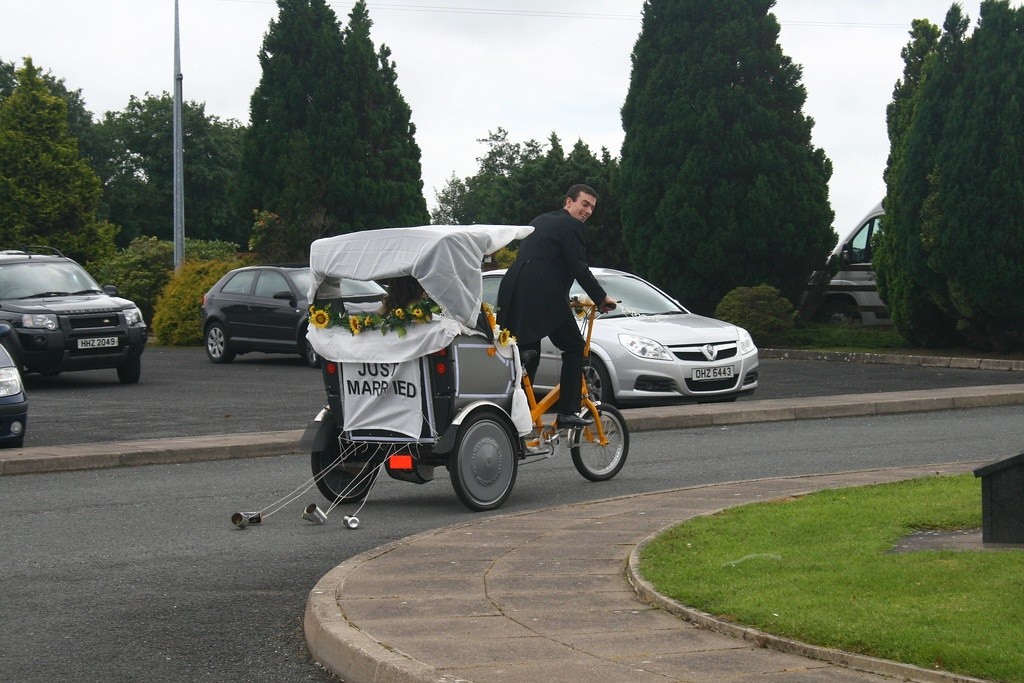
[479,265,760,409]
[0,321,29,451]
[201,263,390,368]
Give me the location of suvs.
[0,245,149,385]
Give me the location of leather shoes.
[555,414,594,429]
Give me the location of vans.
[798,198,896,328]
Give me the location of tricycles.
[300,225,629,513]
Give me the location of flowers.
[572,297,590,316]
[306,297,511,348]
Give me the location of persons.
[496,183,617,428]
[377,276,427,317]
